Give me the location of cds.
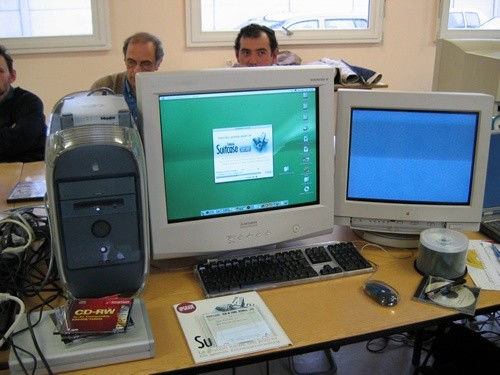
[416,232,465,277]
[425,281,476,307]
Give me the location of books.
[173,290,292,365]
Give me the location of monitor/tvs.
[134,65,335,261]
[332,86,495,248]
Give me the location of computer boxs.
[46,87,149,307]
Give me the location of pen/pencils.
[492,244,500,256]
[425,279,466,296]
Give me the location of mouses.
[364,279,399,307]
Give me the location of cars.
[234,10,369,31]
[446,8,500,31]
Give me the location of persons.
[0,44,47,163]
[234,23,279,68]
[90,32,164,131]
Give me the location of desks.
[0,161,48,211]
[0,220,500,375]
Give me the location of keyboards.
[192,241,378,299]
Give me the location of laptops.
[481,131,500,245]
